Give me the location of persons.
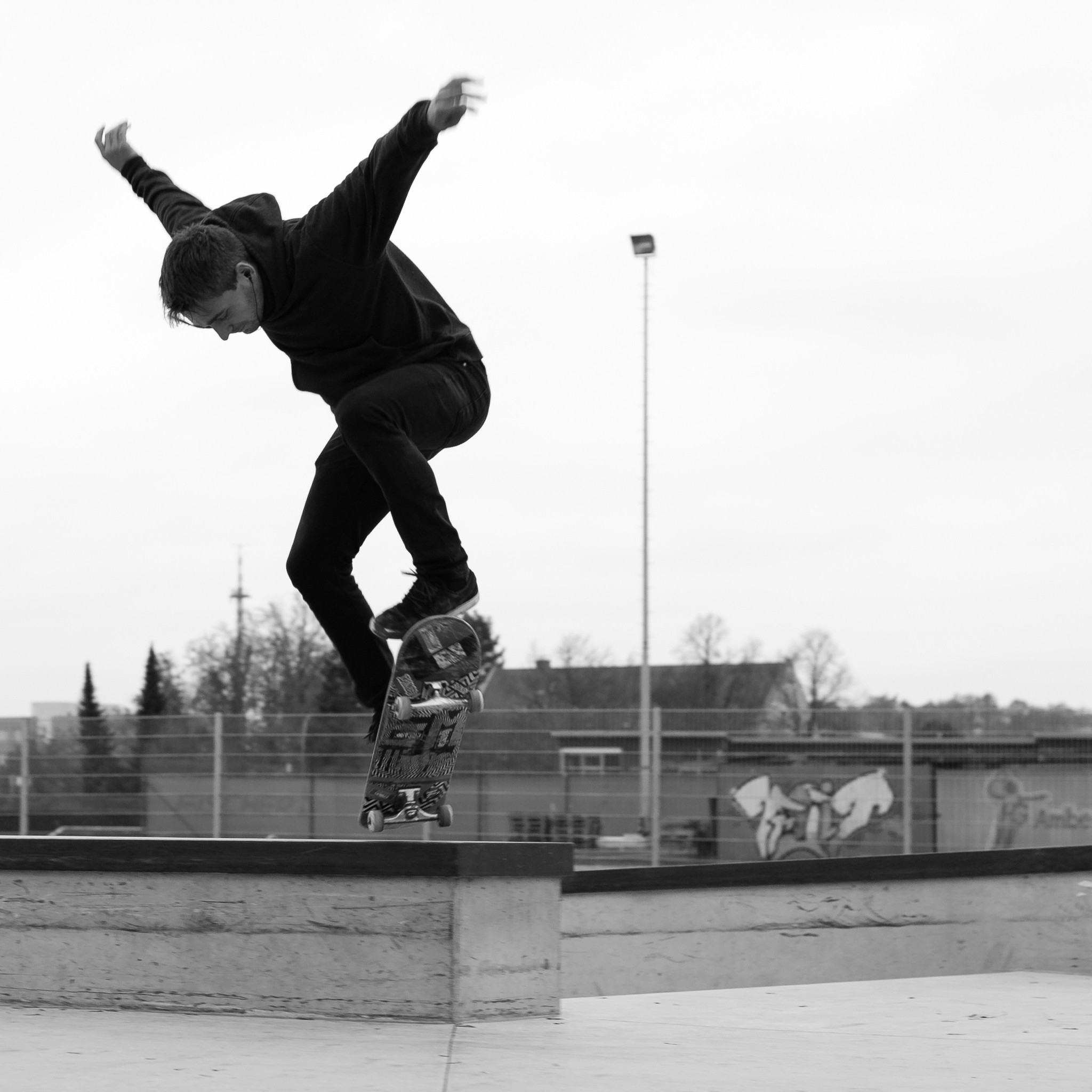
[683,820,717,859]
[93,74,492,743]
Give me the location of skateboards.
[357,614,485,833]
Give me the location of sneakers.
[368,567,480,639]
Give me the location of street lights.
[632,232,657,837]
